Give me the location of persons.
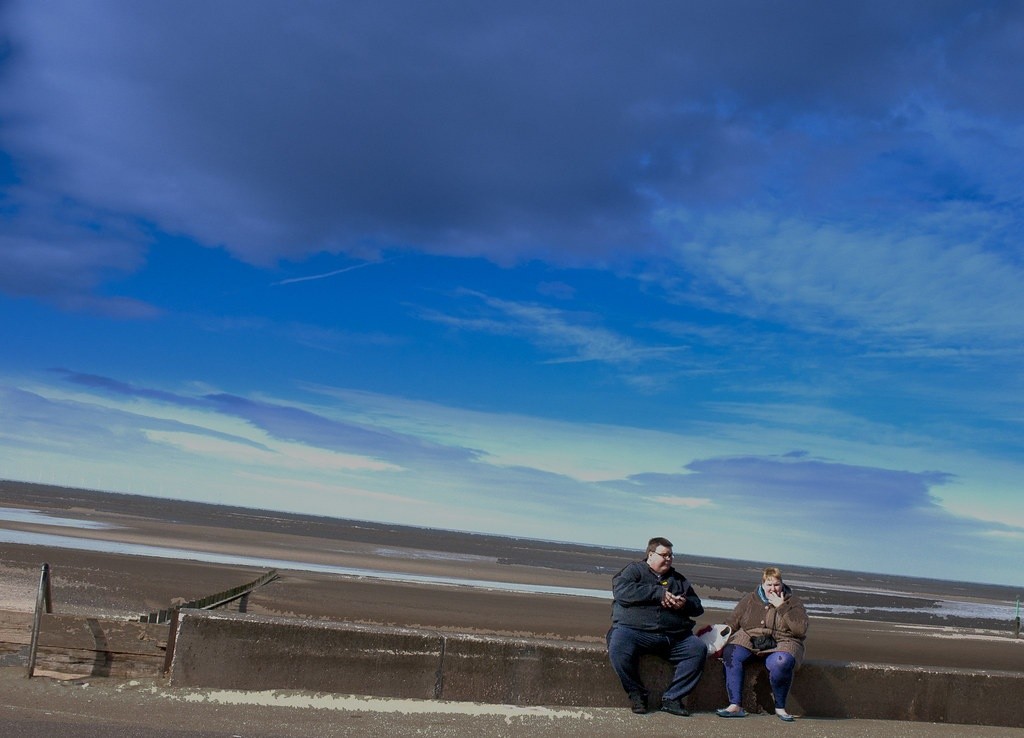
[716,567,809,722]
[606,537,708,718]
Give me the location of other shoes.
[777,712,793,721]
[717,709,745,717]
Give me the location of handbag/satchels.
[750,634,777,651]
[696,624,732,659]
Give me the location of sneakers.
[630,695,647,713]
[658,698,689,717]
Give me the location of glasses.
[653,551,674,560]
